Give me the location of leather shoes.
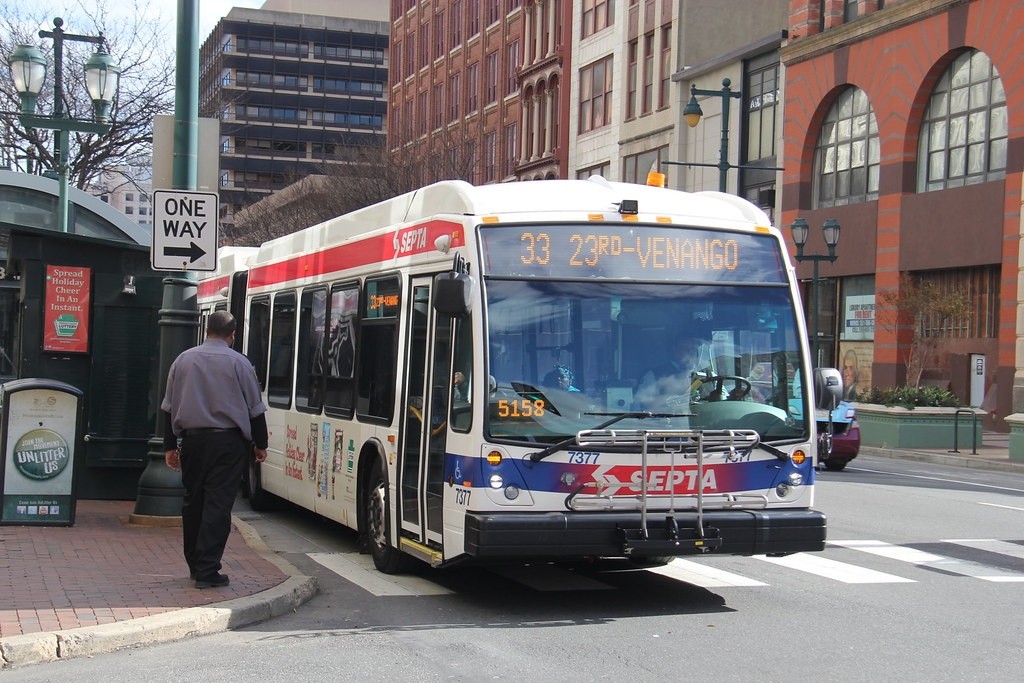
[195,571,229,588]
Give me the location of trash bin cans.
[0,378,85,528]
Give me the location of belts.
[183,427,231,436]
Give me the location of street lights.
[660,76,786,193]
[789,215,841,370]
[7,16,122,235]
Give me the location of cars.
[726,360,860,472]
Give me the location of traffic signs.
[149,190,220,273]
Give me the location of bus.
[197,176,847,576]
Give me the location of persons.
[634,337,729,411]
[161,310,269,588]
[544,368,580,392]
[453,372,467,401]
